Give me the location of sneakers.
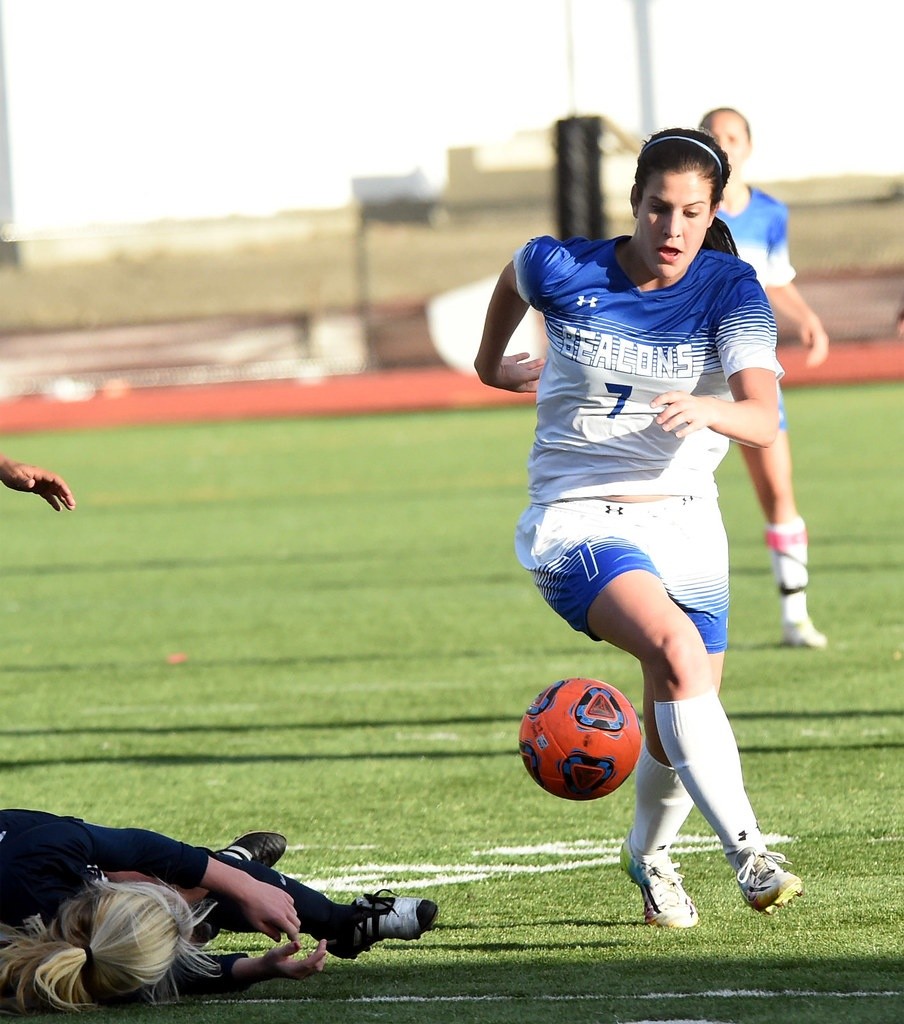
[323,892,437,959]
[619,829,698,927]
[734,845,803,914]
[213,830,287,868]
[787,618,828,648]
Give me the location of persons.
[699,107,828,649]
[474,129,803,930]
[0,808,437,1014]
[0,453,76,512]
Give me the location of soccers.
[518,677,644,801]
